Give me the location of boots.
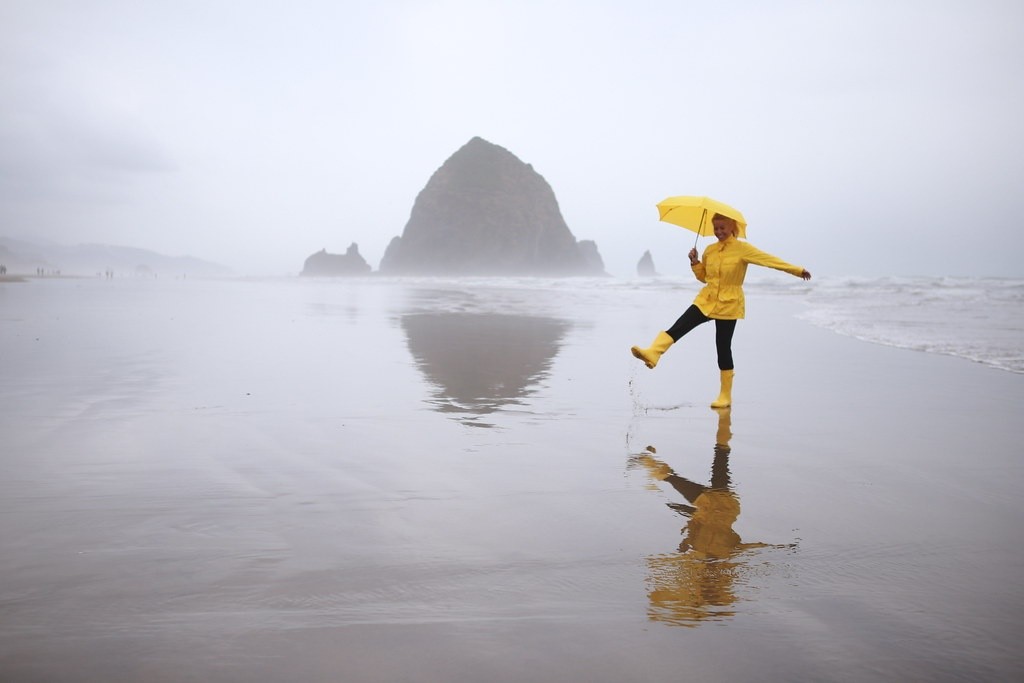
[633,331,674,368]
[711,369,734,409]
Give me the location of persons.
[630,212,812,409]
[626,408,798,560]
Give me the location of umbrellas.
[657,195,748,248]
